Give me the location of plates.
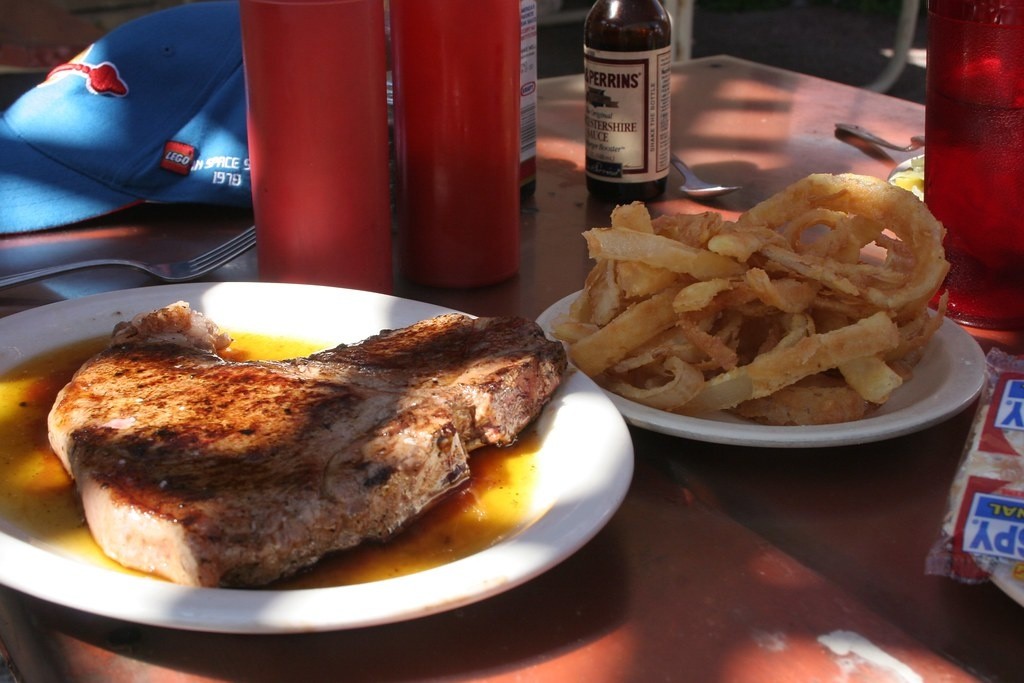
[887,154,924,202]
[0,282,636,633]
[534,288,987,448]
[951,478,1024,609]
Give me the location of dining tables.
[0,55,1024,683]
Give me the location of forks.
[0,225,257,291]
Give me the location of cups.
[383,1,394,107]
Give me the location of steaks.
[46,301,565,590]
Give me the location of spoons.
[670,153,743,200]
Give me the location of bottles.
[924,0,1024,330]
[582,1,671,204]
[243,0,537,294]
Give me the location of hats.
[0,1,252,236]
